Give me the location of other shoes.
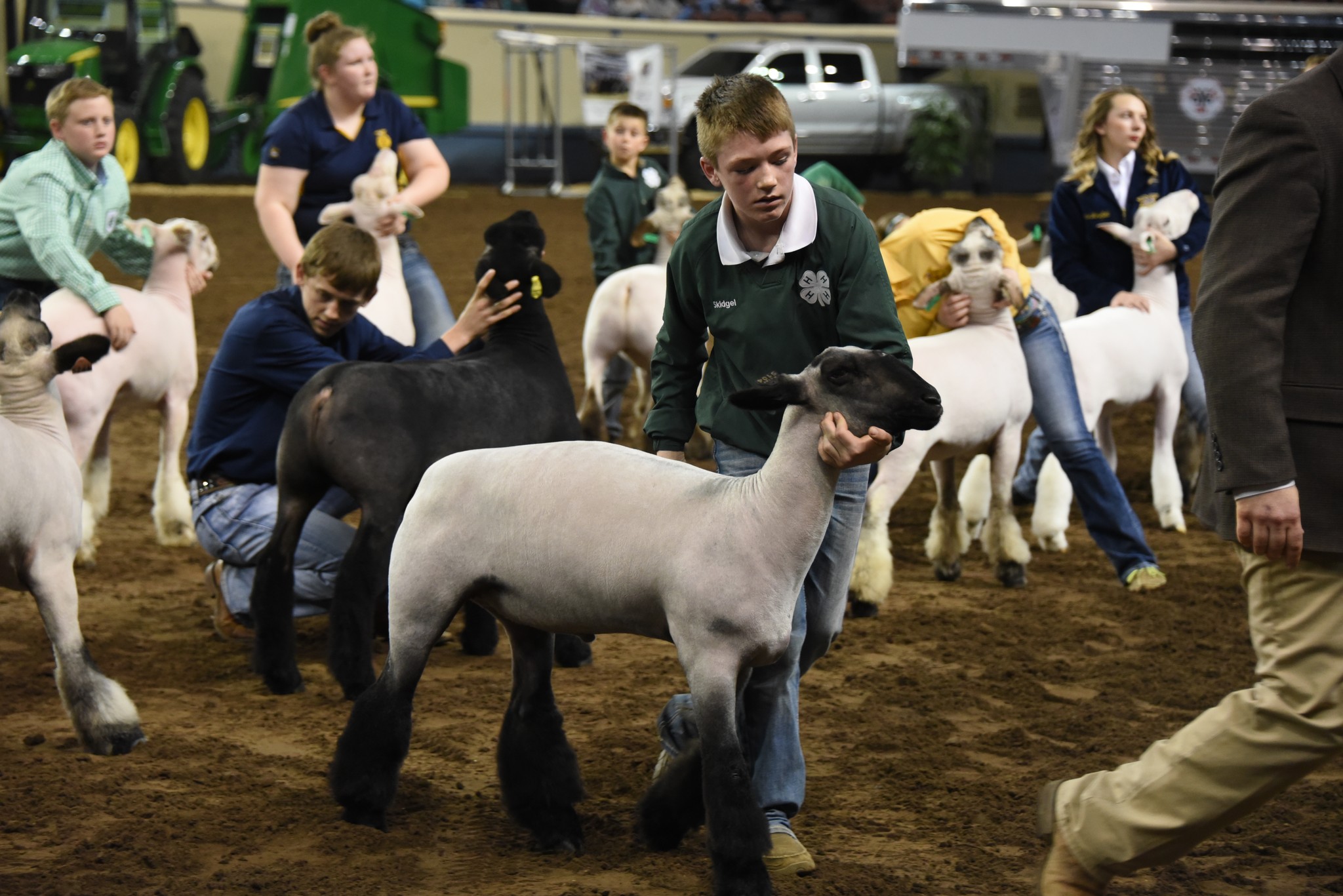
[372,619,455,647]
[1037,779,1107,896]
[205,559,260,645]
[652,749,678,782]
[1010,487,1033,523]
[762,832,817,877]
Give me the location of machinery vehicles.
[1,0,224,188]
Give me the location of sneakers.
[1126,567,1167,593]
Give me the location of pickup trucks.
[648,36,962,195]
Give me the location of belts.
[190,475,235,506]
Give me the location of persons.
[873,86,1209,592]
[1,76,215,353]
[185,224,522,645]
[1036,44,1343,896]
[642,75,913,876]
[252,10,456,354]
[585,101,672,443]
[1304,52,1324,74]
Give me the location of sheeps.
[249,208,598,693]
[0,288,145,757]
[959,188,1200,549]
[329,342,945,896]
[318,148,416,349]
[845,220,1032,621]
[577,258,714,432]
[37,215,220,545]
